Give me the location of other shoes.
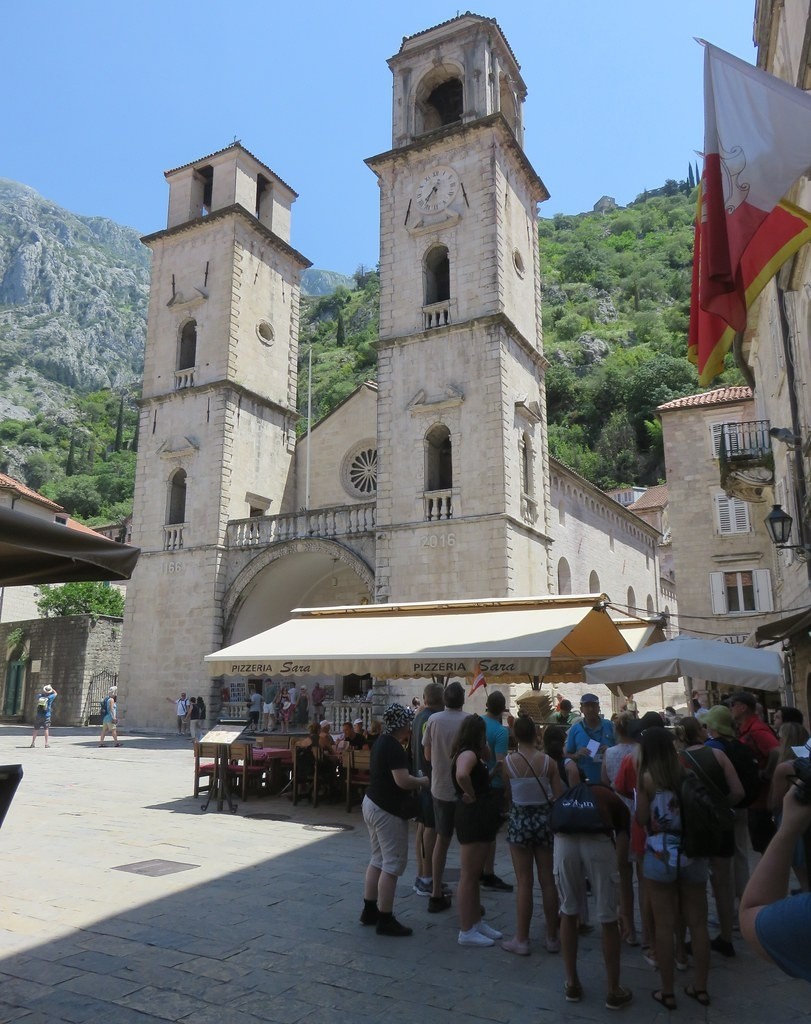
[114,743,124,747]
[98,744,106,747]
[45,745,50,748]
[30,744,36,747]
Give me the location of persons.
[297,719,382,803]
[166,692,205,742]
[411,683,811,1011]
[360,702,429,937]
[245,677,326,733]
[30,684,58,748]
[99,686,123,747]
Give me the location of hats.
[337,740,350,752]
[580,694,599,704]
[109,686,117,693]
[697,705,734,736]
[319,720,333,728]
[283,702,292,710]
[791,736,811,758]
[43,685,52,693]
[354,718,364,724]
[724,692,756,710]
[383,703,415,734]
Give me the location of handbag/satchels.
[101,698,109,715]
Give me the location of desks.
[250,748,291,798]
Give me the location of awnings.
[204,592,668,681]
[743,608,811,648]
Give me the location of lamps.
[762,504,808,561]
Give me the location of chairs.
[194,738,371,813]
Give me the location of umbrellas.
[583,631,786,716]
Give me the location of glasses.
[731,702,743,707]
[300,689,305,690]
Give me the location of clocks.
[414,165,460,216]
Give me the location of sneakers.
[360,865,741,1010]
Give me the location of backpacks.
[38,694,48,711]
[547,782,605,836]
[671,768,736,859]
[713,738,756,806]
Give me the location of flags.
[688,40,811,387]
